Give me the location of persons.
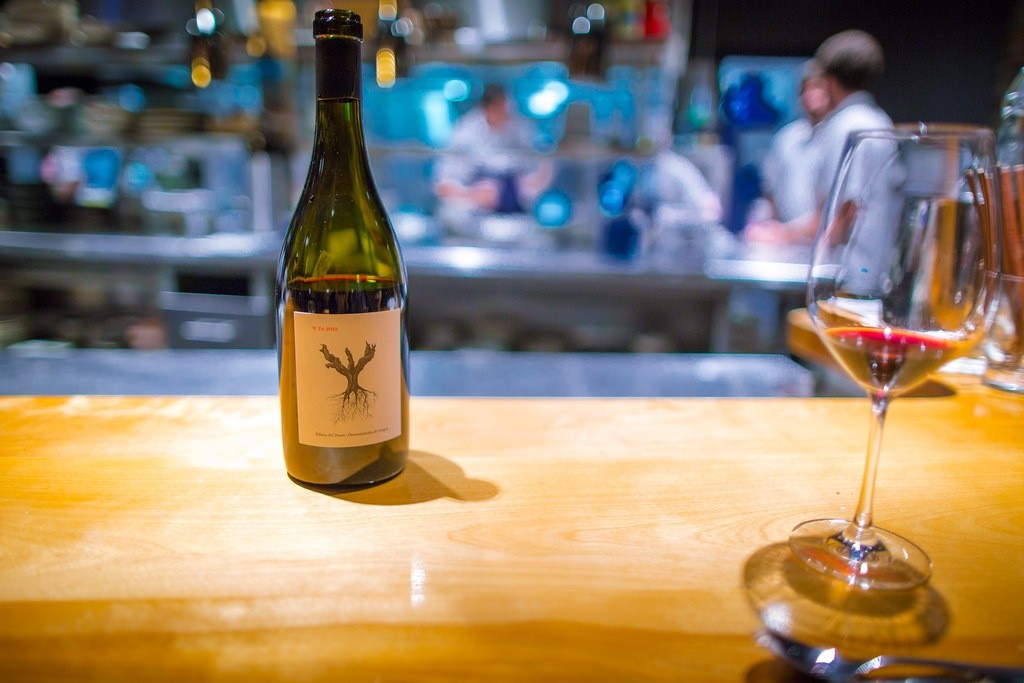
[430,25,910,369]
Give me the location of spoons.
[755,627,1024,683]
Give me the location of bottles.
[275,9,409,485]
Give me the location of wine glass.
[787,124,1001,593]
